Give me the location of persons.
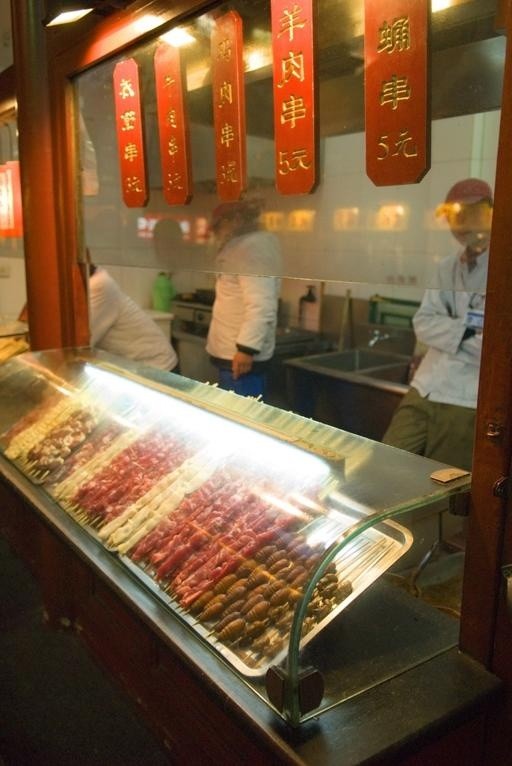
[204,199,281,404]
[87,248,181,376]
[379,177,494,471]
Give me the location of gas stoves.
[167,292,222,325]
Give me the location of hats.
[436,179,490,217]
[203,203,248,240]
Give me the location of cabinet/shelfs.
[2,345,510,764]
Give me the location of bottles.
[153,270,173,312]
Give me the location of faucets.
[368,329,392,348]
[298,284,317,304]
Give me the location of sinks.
[348,361,416,394]
[283,348,410,374]
